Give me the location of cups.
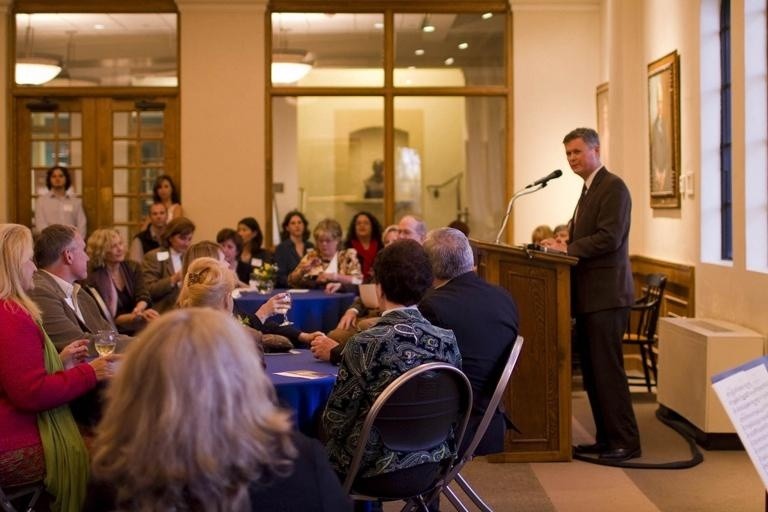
[95,330,117,357]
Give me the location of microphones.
[525,169,563,189]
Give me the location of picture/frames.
[647,49,679,208]
[596,82,610,133]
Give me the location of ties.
[578,184,586,209]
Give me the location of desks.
[234,288,355,348]
[74,348,340,438]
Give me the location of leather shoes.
[576,441,606,454]
[599,444,642,462]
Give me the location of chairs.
[343,361,473,512]
[622,274,666,393]
[0,479,44,512]
[401,335,524,512]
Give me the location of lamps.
[15,26,63,85]
[271,48,313,84]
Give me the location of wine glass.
[279,293,294,326]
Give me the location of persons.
[2,201,518,510]
[533,128,642,462]
[363,159,384,198]
[34,166,87,240]
[141,175,183,232]
[651,83,670,191]
